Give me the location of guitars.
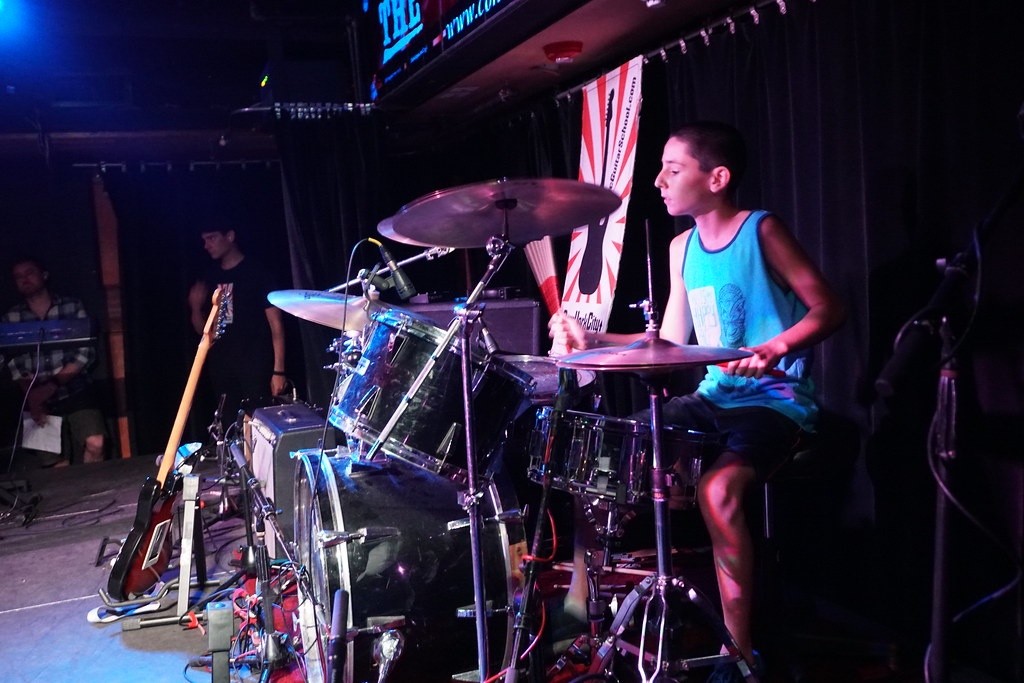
[104,280,231,602]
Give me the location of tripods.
[122,393,332,683]
[543,366,757,683]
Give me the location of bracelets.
[51,377,61,388]
[272,371,284,376]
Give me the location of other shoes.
[706,648,767,683]
[550,604,594,653]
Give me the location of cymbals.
[265,286,386,333]
[554,325,755,377]
[375,176,622,248]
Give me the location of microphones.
[378,244,416,299]
[330,589,349,683]
[477,316,501,355]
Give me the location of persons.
[541,108,844,683]
[190,215,285,485]
[0,256,107,466]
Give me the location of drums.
[291,446,519,682]
[325,301,540,496]
[521,408,717,512]
[488,350,601,471]
